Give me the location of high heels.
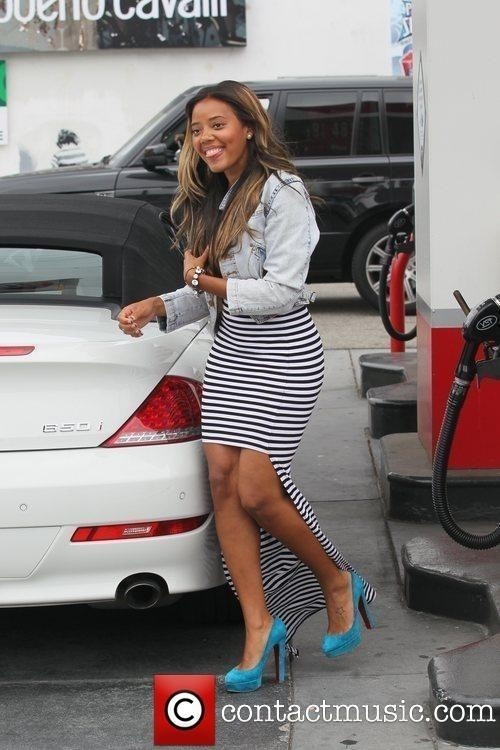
[318,571,375,659]
[223,614,291,694]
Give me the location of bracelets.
[184,267,196,284]
[192,267,206,294]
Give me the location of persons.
[114,78,379,695]
